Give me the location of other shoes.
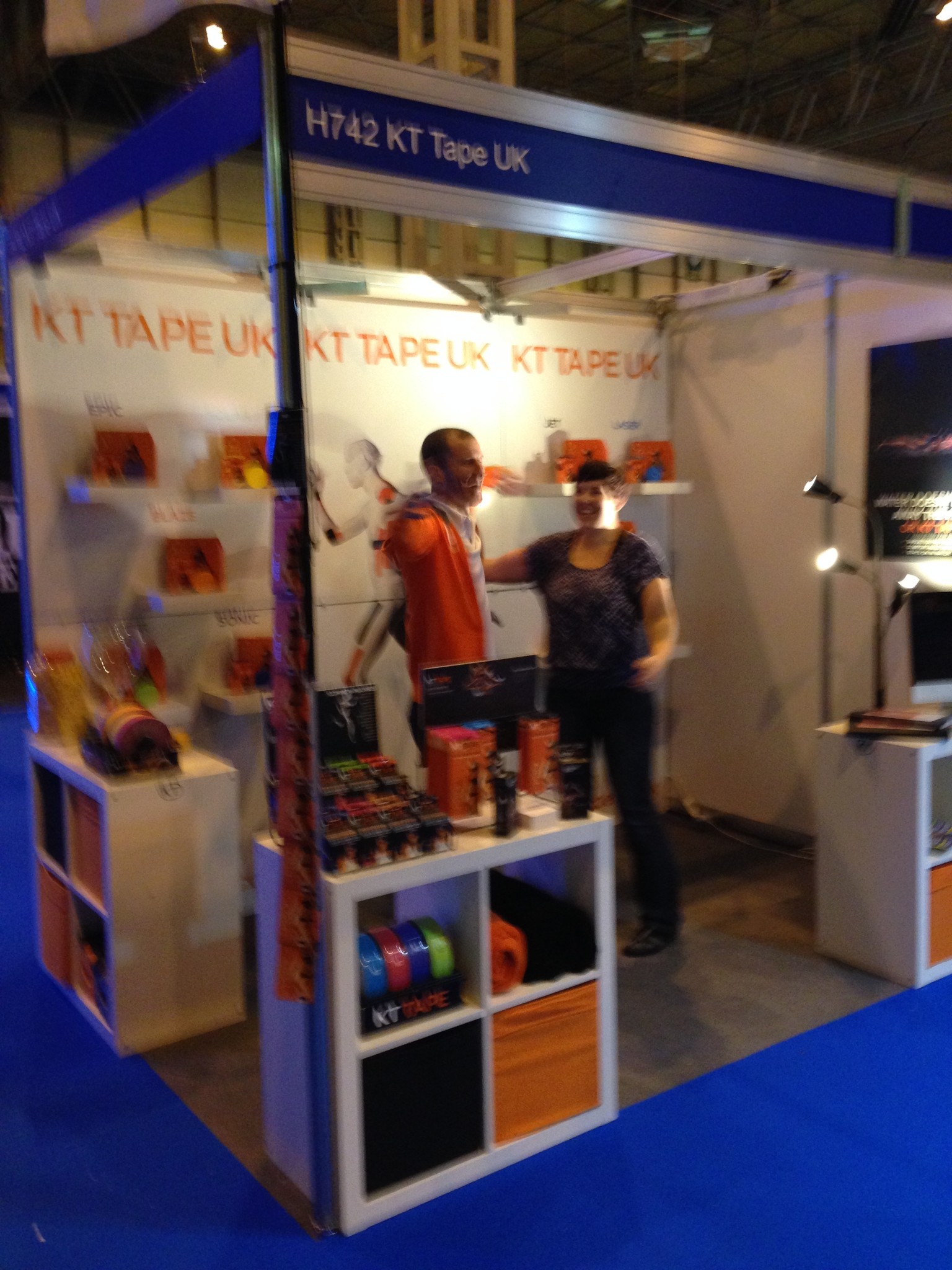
[621,928,675,956]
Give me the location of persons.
[381,428,491,782]
[481,459,683,957]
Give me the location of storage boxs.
[516,716,561,798]
[423,719,500,820]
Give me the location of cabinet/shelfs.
[807,716,952,993]
[26,464,696,1245]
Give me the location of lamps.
[795,471,868,512]
[811,543,877,587]
[878,571,921,641]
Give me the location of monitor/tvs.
[419,651,539,762]
[907,588,952,703]
